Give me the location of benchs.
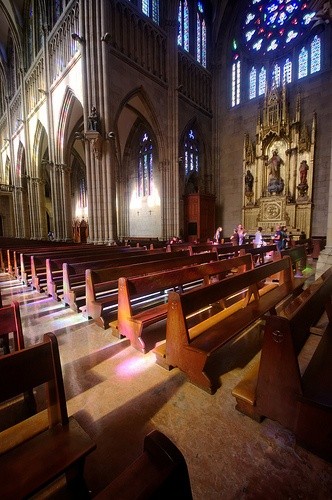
[0,237,332,500]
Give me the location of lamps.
[177,84,184,90]
[71,32,82,43]
[17,118,24,123]
[3,138,10,141]
[107,131,115,138]
[74,131,82,138]
[37,88,46,95]
[103,33,112,41]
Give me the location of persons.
[254,227,263,247]
[215,227,223,243]
[48,232,52,238]
[230,225,246,246]
[271,225,290,250]
[299,161,308,183]
[265,151,284,177]
[164,236,183,249]
[245,170,254,192]
